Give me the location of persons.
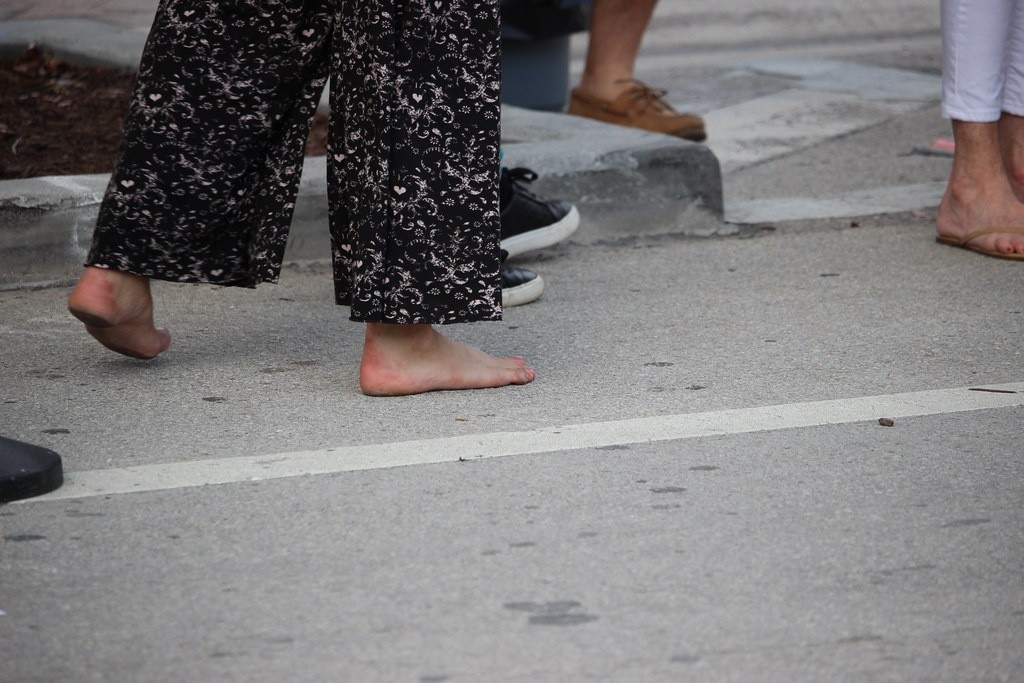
[69,0,536,396]
[935,0,1024,260]
[499,166,580,306]
[498,1,706,140]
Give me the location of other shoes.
[0,435,64,505]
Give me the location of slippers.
[934,214,1024,260]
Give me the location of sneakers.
[498,264,542,306]
[494,166,581,259]
[568,76,705,138]
